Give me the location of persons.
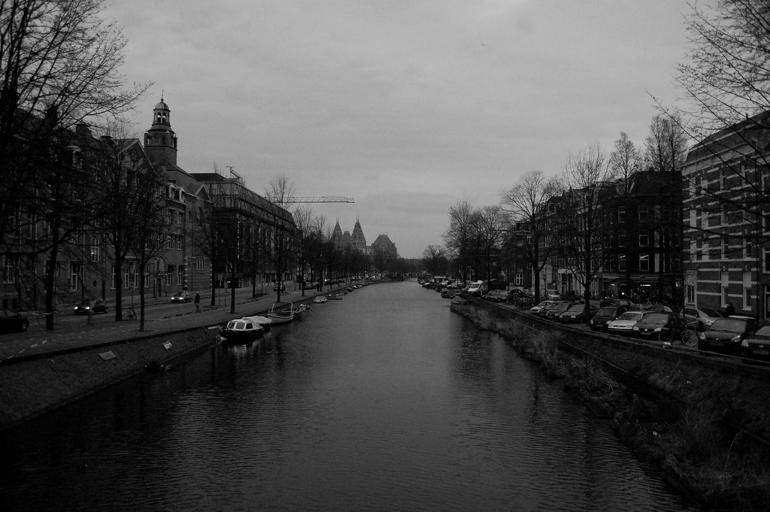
[726,302,734,312]
[194,292,201,313]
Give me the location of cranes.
[264,193,355,204]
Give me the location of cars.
[0,308,30,333]
[170,291,194,303]
[273,283,286,291]
[304,270,379,289]
[74,297,109,315]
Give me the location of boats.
[207,301,311,340]
[312,281,369,303]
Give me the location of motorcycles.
[123,306,138,322]
